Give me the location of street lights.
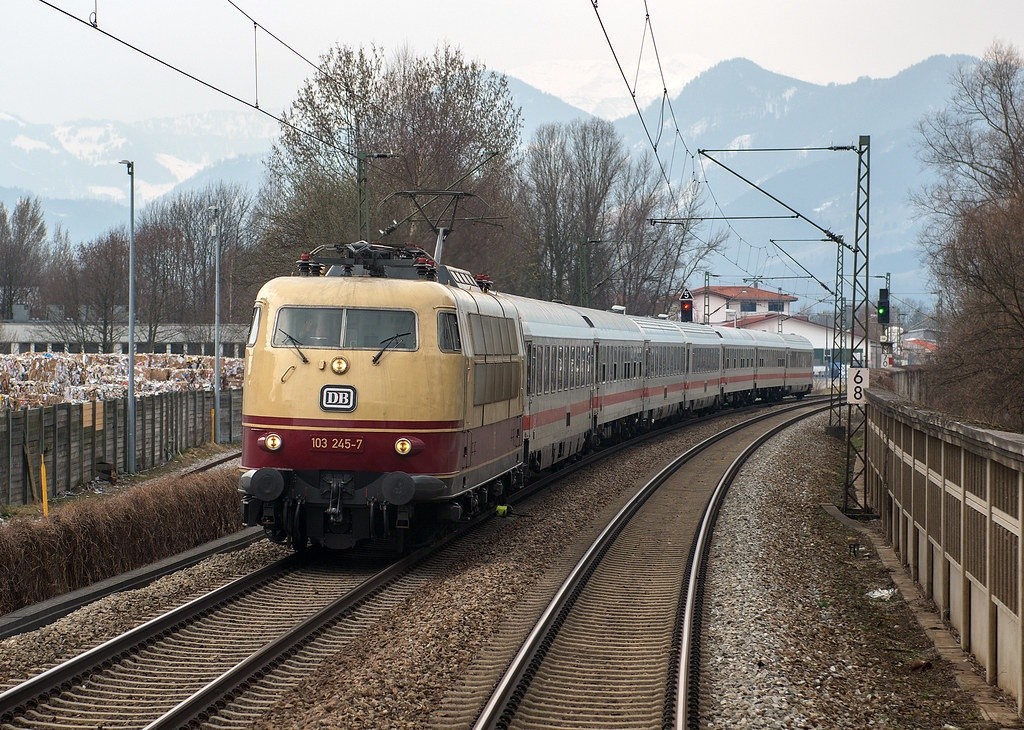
[119,159,136,474]
[208,206,220,445]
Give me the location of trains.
[240,190,814,559]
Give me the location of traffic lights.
[878,300,889,323]
[682,300,692,322]
